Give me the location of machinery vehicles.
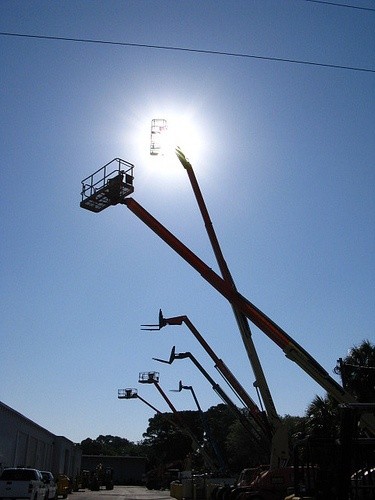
[77,118,375,499]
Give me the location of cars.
[39,470,73,500]
[0,468,50,500]
